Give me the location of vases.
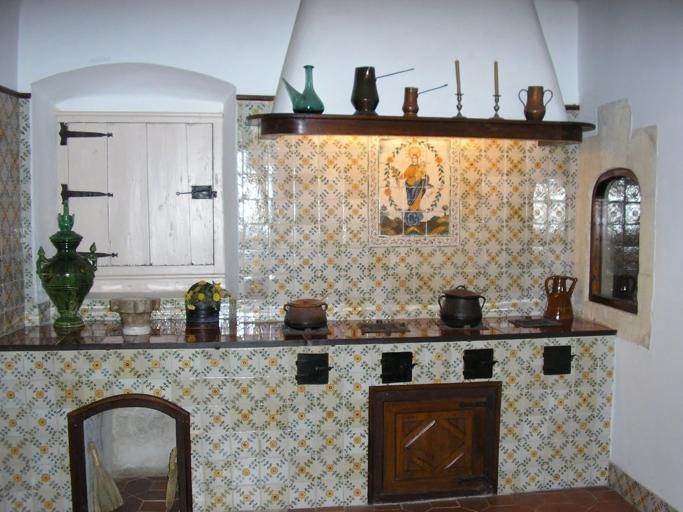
[187,307,219,333]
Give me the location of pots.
[437,287,486,328]
[282,300,327,330]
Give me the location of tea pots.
[545,275,578,323]
[611,272,635,300]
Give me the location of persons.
[400,153,426,212]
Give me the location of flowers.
[184,280,234,315]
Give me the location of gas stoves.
[284,316,571,385]
